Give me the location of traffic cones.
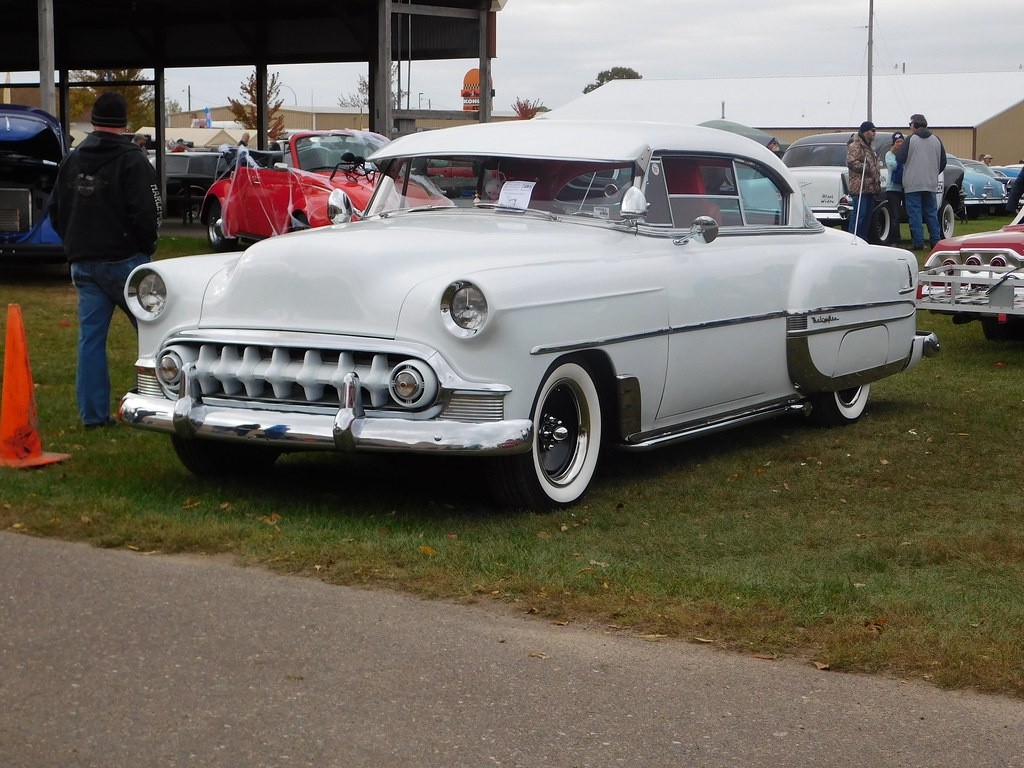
[1,303,72,469]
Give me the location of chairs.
[298,147,330,170]
[645,160,722,228]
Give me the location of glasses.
[870,130,875,132]
[909,122,914,127]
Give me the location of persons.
[51,92,164,428]
[767,114,995,251]
[131,132,280,156]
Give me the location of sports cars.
[205,129,456,251]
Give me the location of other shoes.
[907,245,924,251]
[83,419,117,430]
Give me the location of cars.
[149,149,231,220]
[780,130,1024,241]
[0,104,74,266]
[916,203,1024,342]
[120,117,919,513]
[550,121,777,220]
[408,157,508,198]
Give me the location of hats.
[135,134,148,140]
[891,131,904,143]
[983,155,994,159]
[860,121,875,133]
[91,93,128,128]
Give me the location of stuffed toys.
[483,172,503,200]
